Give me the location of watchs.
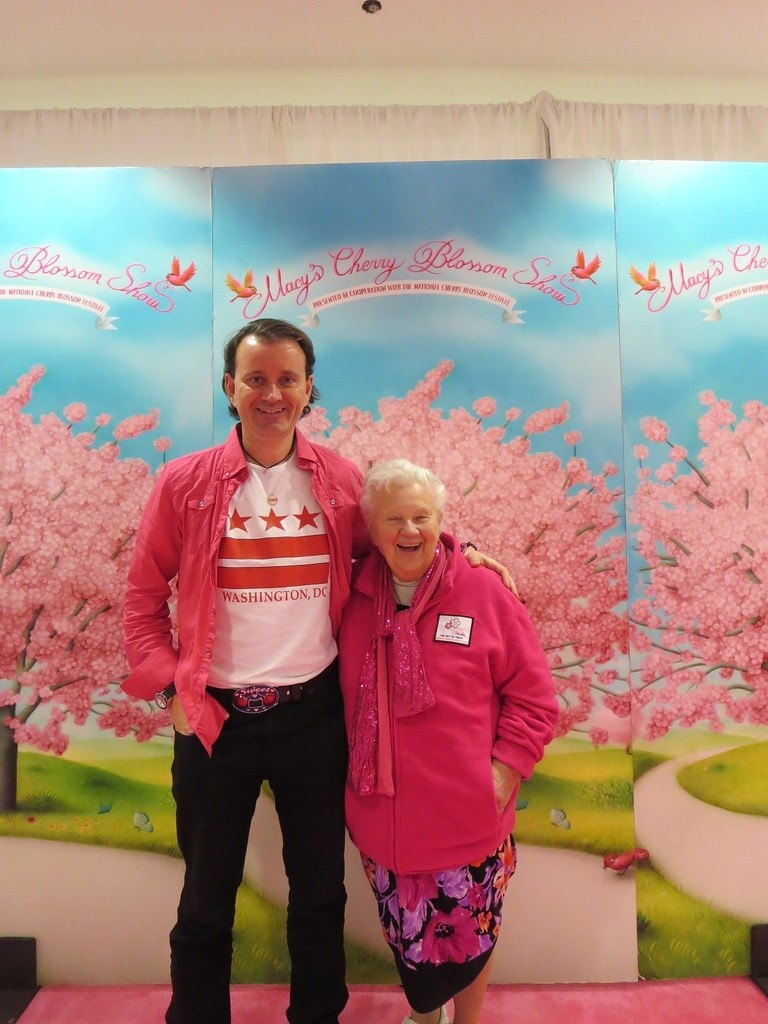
[154,681,177,710]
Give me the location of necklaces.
[243,450,288,505]
[241,437,294,478]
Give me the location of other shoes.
[402,1004,449,1024]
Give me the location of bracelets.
[460,542,478,554]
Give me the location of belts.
[207,655,339,714]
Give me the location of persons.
[123,318,520,1024]
[339,460,560,1024]
